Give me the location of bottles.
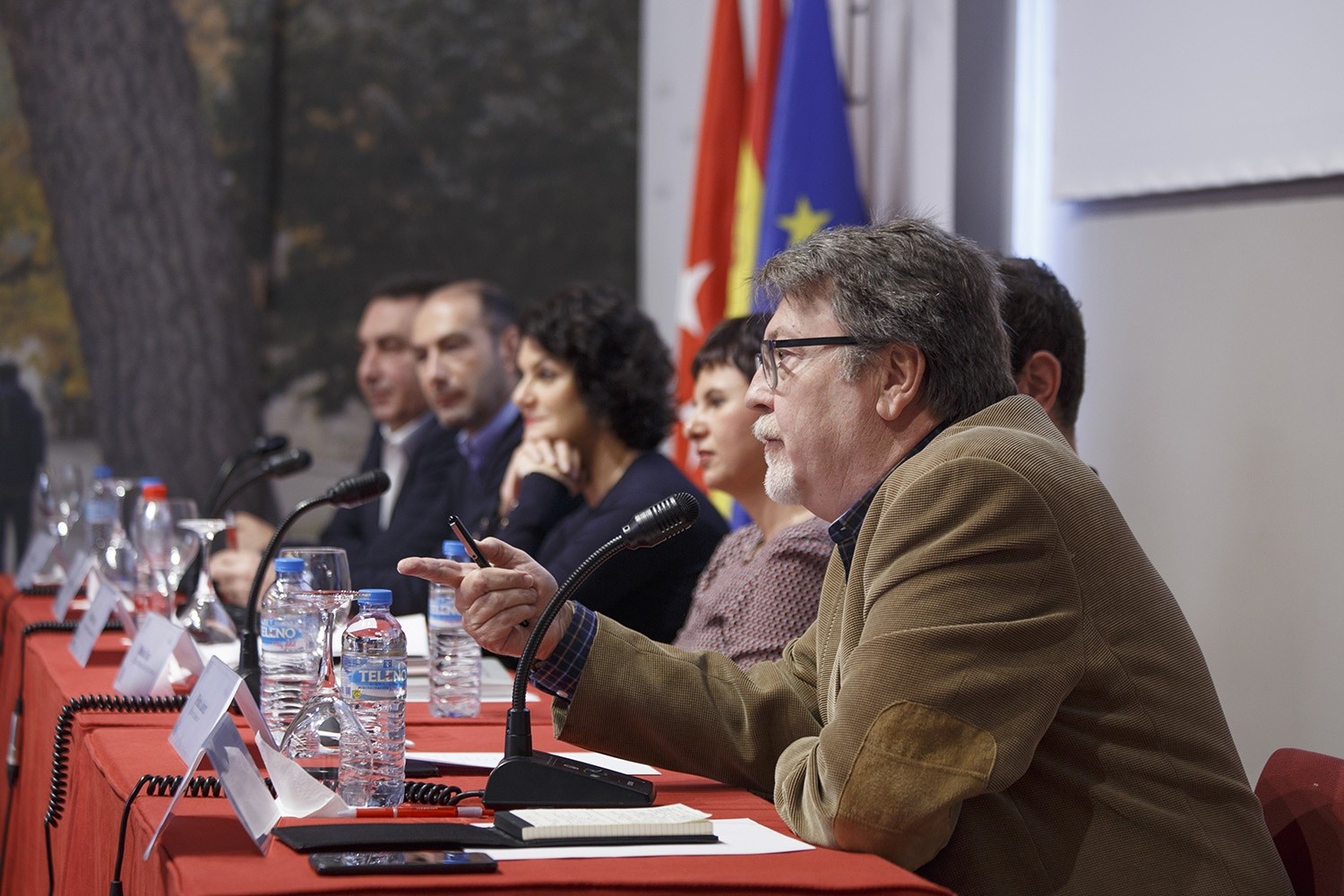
[129,483,178,624]
[340,589,407,810]
[428,541,481,719]
[87,465,118,566]
[258,557,322,757]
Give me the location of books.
[494,803,714,842]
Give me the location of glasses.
[755,335,932,389]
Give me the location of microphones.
[183,449,311,629]
[179,435,286,591]
[228,468,393,713]
[481,492,700,806]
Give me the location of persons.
[209,279,447,621]
[989,258,1086,448]
[0,365,46,574]
[395,217,1297,895]
[470,280,732,643]
[408,273,527,556]
[670,312,834,670]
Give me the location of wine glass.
[176,519,241,671]
[36,460,142,645]
[134,497,200,622]
[279,546,373,812]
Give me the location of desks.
[0,568,955,896]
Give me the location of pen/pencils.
[445,513,530,628]
[302,767,441,780]
[337,804,499,816]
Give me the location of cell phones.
[310,850,497,877]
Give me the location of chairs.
[1251,742,1344,896]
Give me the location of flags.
[672,0,744,496]
[733,0,867,529]
[705,0,788,517]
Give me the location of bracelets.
[566,600,575,615]
